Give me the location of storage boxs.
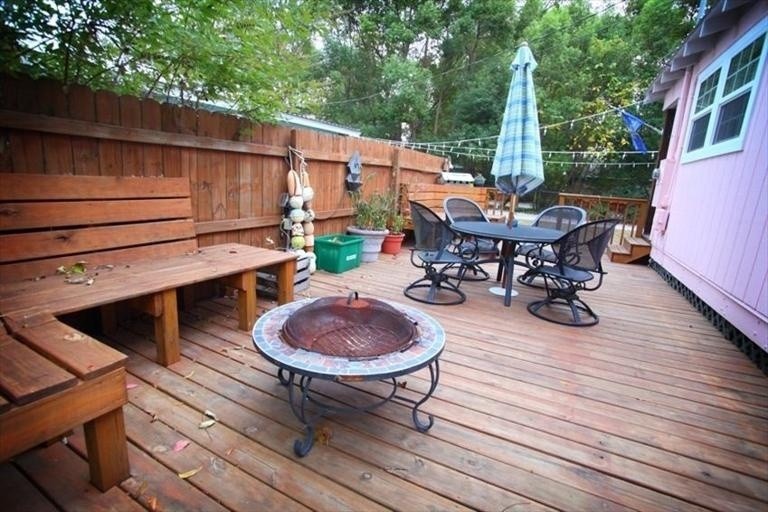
[314,234,364,274]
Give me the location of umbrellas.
[490,41,545,229]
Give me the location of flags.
[616,108,647,154]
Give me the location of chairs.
[403,196,620,327]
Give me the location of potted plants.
[382,189,405,255]
[346,173,391,263]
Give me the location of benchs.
[0,172,301,492]
[399,183,487,249]
